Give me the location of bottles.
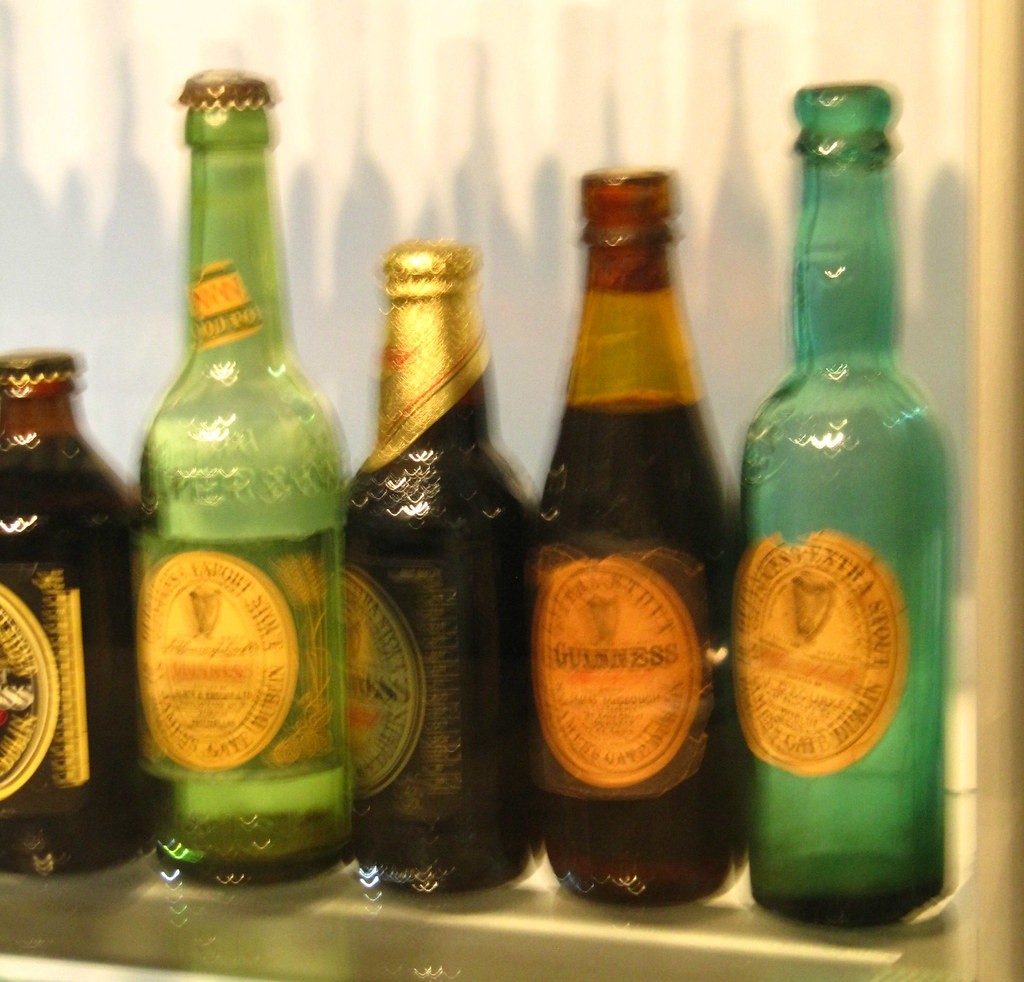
[335,239,541,894]
[132,72,357,888]
[529,168,749,909]
[739,80,952,929]
[0,351,152,878]
